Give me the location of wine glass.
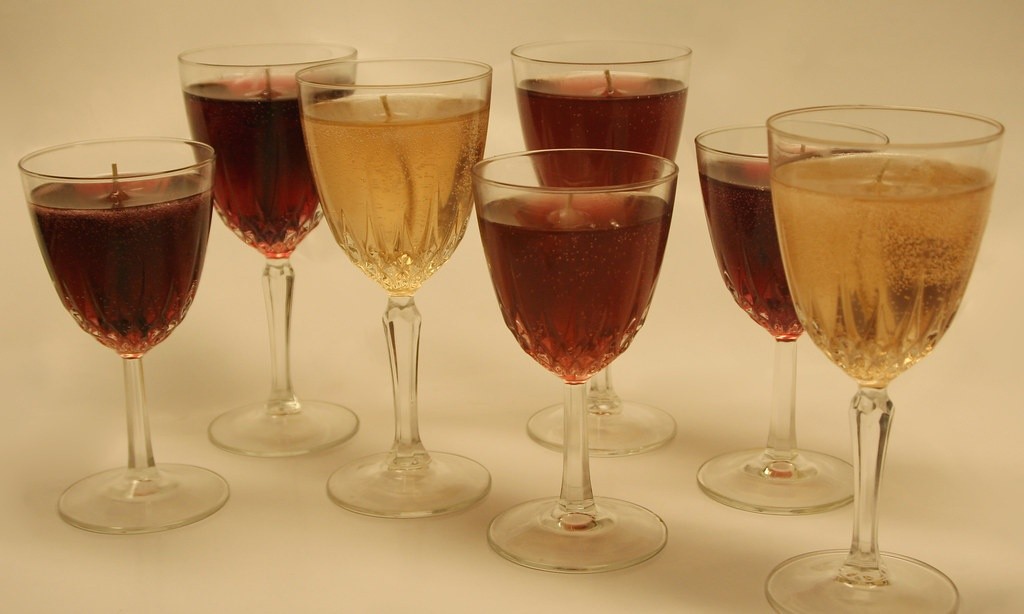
[293,57,496,520]
[179,46,364,459]
[473,154,672,576]
[16,136,233,535]
[510,38,692,458]
[764,104,1003,614]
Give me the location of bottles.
[691,120,894,516]
